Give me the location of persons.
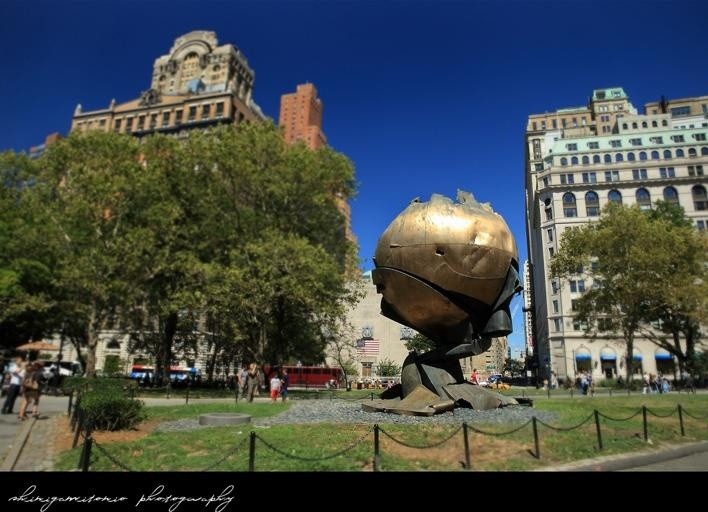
[1,362,696,404]
[18,357,48,421]
[2,356,26,415]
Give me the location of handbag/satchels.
[23,372,39,390]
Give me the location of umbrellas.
[19,338,63,355]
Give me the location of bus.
[130,364,197,390]
[35,359,82,380]
[262,365,345,390]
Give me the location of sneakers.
[18,415,27,421]
[32,411,40,416]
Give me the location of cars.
[485,381,511,391]
